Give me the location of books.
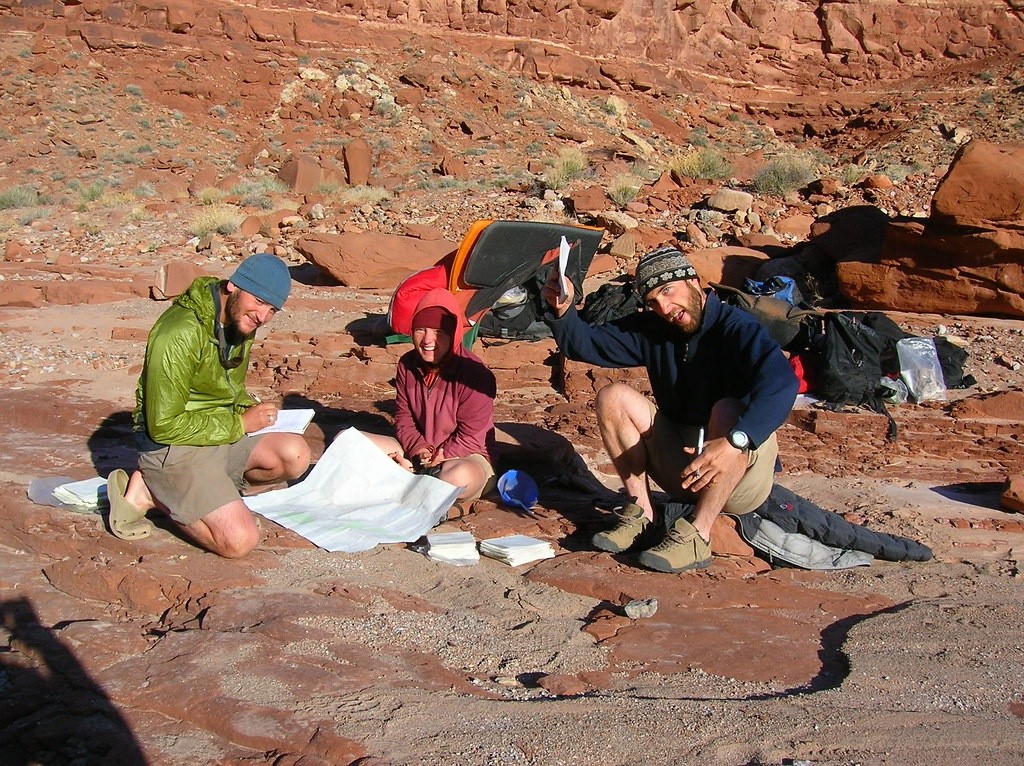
[558,235,571,304]
[247,409,315,437]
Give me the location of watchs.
[727,429,750,453]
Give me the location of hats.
[411,305,456,333]
[636,245,700,300]
[229,253,291,310]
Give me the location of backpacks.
[742,273,804,306]
[579,282,651,328]
[800,308,886,413]
[479,294,539,347]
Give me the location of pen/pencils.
[695,426,705,479]
[250,392,264,404]
[419,457,460,465]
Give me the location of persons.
[333,289,500,501]
[106,254,311,559]
[541,244,800,573]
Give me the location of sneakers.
[591,496,662,553]
[637,518,713,574]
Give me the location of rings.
[269,414,274,422]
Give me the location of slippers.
[106,469,152,540]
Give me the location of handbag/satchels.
[895,336,947,402]
